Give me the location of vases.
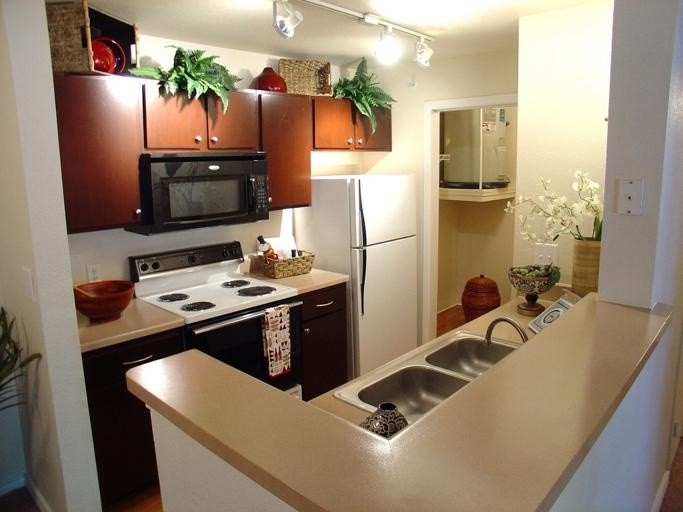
[569,237,601,295]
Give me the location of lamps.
[271,0,435,68]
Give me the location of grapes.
[512,264,551,277]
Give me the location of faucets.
[483,316,527,348]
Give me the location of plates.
[92,37,127,75]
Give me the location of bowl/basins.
[73,279,138,324]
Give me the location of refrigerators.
[289,173,418,377]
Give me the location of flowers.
[506,180,604,240]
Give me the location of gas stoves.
[135,276,297,325]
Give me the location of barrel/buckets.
[460,274,501,322]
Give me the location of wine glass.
[507,267,558,316]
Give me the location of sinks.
[333,364,469,423]
[425,328,522,380]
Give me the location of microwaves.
[123,150,270,238]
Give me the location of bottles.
[250,65,289,93]
[357,400,408,438]
[256,233,278,261]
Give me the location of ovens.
[192,300,301,403]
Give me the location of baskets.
[44,0,140,76]
[258,250,315,279]
[279,58,333,97]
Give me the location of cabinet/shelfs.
[143,80,259,152]
[54,72,143,235]
[298,282,348,398]
[311,95,392,150]
[81,324,188,509]
[257,92,310,214]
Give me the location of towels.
[261,304,291,377]
